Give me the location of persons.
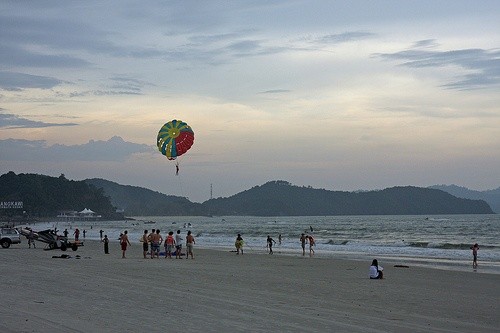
[103,235,110,254]
[369,259,383,279]
[185,231,195,259]
[235,234,244,255]
[28,229,36,248]
[83,230,85,238]
[55,227,59,234]
[300,233,315,255]
[267,235,276,253]
[72,229,79,241]
[310,226,313,232]
[279,235,281,244]
[176,165,179,175]
[470,243,479,269]
[63,229,69,236]
[120,231,131,258]
[143,229,183,259]
[99,230,104,242]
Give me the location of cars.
[0,228,22,248]
